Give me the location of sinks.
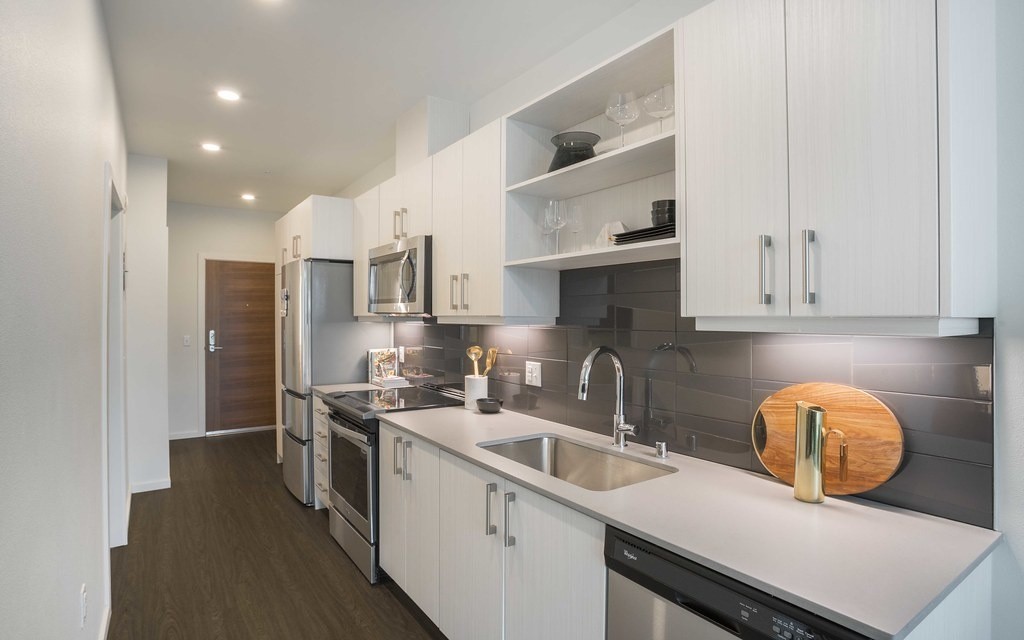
[474,432,680,492]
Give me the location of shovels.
[482,348,497,376]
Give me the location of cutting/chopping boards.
[751,382,905,495]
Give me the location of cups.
[465,375,488,409]
[499,373,521,408]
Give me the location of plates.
[613,223,676,245]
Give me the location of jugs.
[794,400,848,504]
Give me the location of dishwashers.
[603,523,874,640]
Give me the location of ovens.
[324,411,379,584]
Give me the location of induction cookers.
[321,383,465,419]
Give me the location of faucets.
[643,343,698,437]
[576,345,642,448]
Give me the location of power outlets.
[526,361,541,387]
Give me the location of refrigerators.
[281,258,394,506]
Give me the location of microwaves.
[368,234,432,314]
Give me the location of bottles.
[547,131,601,173]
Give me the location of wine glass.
[605,92,640,147]
[537,208,554,256]
[566,205,586,252]
[641,87,675,135]
[547,199,567,254]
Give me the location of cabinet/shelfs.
[276,0,995,337]
[312,394,331,510]
[379,421,607,640]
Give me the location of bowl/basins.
[651,213,675,226]
[476,398,504,413]
[513,394,537,410]
[651,199,675,209]
[651,207,675,216]
